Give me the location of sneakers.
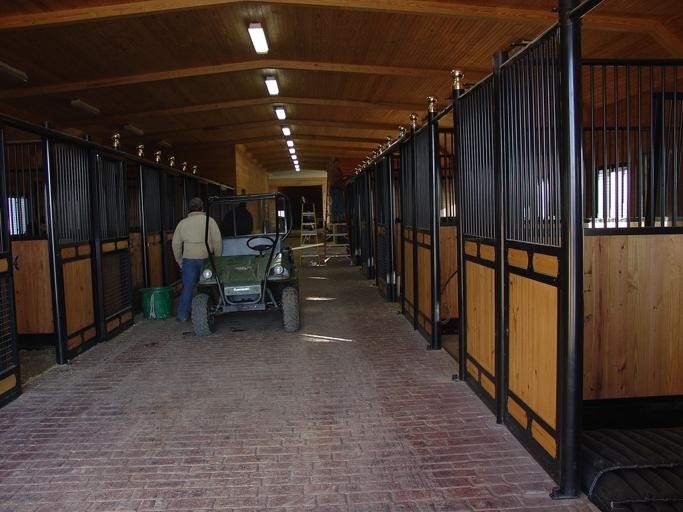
[175,315,190,324]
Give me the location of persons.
[223,202,253,237]
[171,198,223,324]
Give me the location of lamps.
[274,105,286,122]
[264,76,279,97]
[245,22,271,56]
[281,125,300,173]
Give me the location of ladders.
[324,170,352,263]
[300,203,320,265]
